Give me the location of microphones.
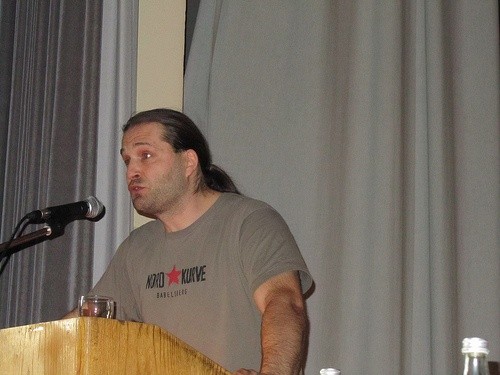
[24,196,103,224]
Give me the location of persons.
[56,108,316,375]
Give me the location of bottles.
[460,337,494,375]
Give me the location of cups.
[77,293,116,320]
